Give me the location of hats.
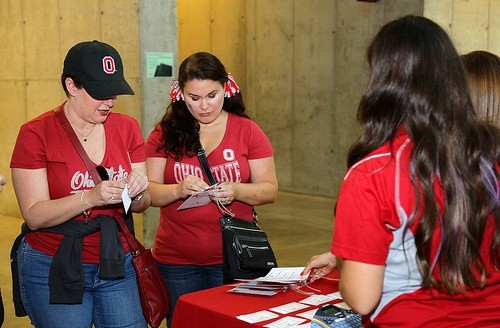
[61,40,136,100]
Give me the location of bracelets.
[80,190,91,223]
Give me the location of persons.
[9,40,152,328]
[300,13,500,328]
[144,52,279,328]
[0,175,7,328]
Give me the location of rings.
[111,193,113,200]
[225,198,228,203]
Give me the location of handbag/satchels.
[220,214,279,284]
[130,246,170,327]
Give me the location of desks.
[172,267,372,328]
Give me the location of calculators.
[310,303,363,328]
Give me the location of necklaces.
[69,120,96,143]
